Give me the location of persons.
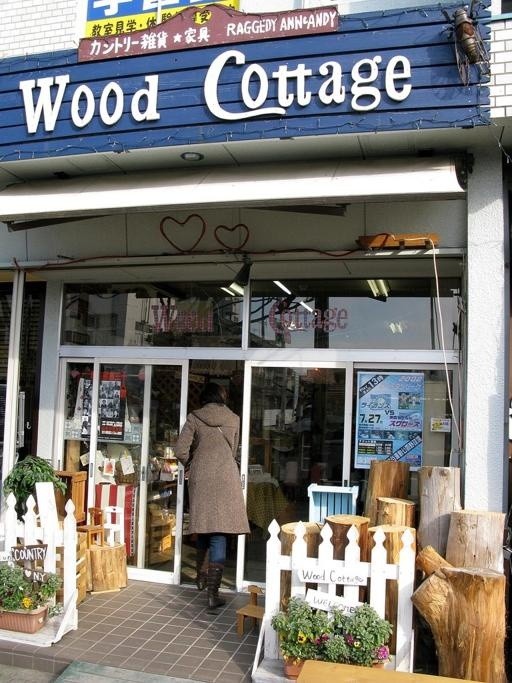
[172,381,252,611]
[83,381,121,435]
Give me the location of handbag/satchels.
[167,480,189,513]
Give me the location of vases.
[367,662,385,671]
[282,654,305,680]
[0,607,48,634]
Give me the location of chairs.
[77,507,104,549]
[236,586,265,636]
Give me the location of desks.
[295,660,485,682]
[249,474,289,543]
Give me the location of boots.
[207,562,225,607]
[197,546,211,590]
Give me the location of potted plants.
[3,454,69,518]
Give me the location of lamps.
[218,283,244,300]
[368,280,388,299]
[389,319,405,336]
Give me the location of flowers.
[272,600,394,666]
[0,565,59,611]
